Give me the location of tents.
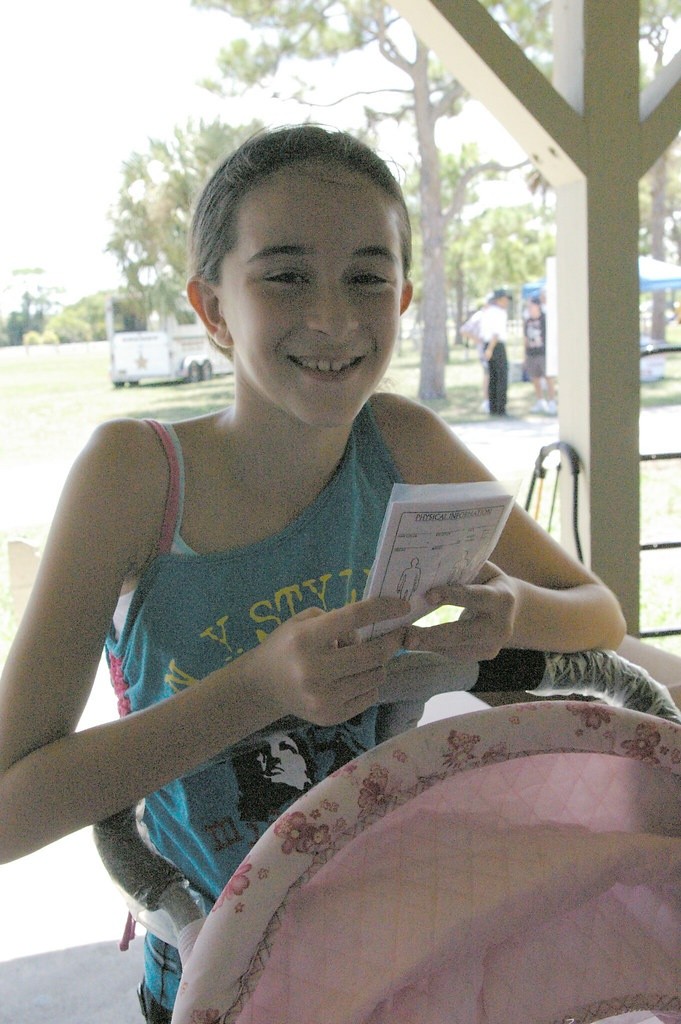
[521,254,681,301]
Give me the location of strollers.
[99,639,681,1024]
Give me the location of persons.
[1,122,627,1024]
[458,287,559,418]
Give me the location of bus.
[107,290,234,388]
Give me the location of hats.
[495,289,512,300]
